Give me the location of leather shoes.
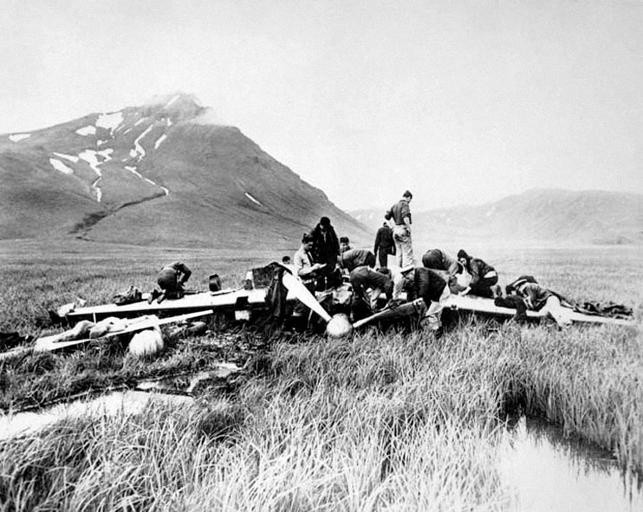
[148,288,157,304]
[157,289,168,303]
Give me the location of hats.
[399,266,413,279]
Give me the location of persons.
[148,262,191,304]
[264,191,572,340]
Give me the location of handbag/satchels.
[112,284,143,305]
